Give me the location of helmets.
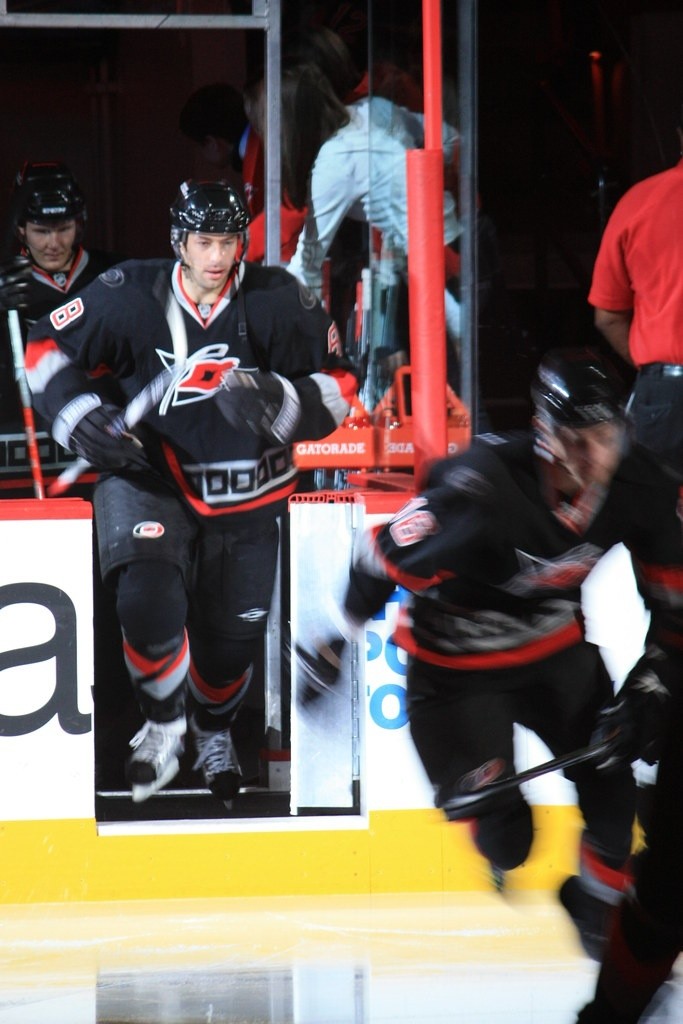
[531,339,626,427]
[170,177,250,233]
[13,162,86,224]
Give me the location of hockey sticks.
[428,720,639,830]
[41,333,238,505]
[5,302,48,508]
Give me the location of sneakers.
[189,714,242,811]
[129,714,187,803]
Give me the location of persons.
[230,23,481,499]
[295,349,683,963]
[25,173,366,809]
[0,159,117,500]
[584,160,682,488]
[558,613,683,1023]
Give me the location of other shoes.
[470,789,533,892]
[558,876,611,963]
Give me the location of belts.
[639,363,683,377]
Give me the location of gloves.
[32,363,144,476]
[587,658,683,776]
[216,371,338,448]
[0,260,35,310]
